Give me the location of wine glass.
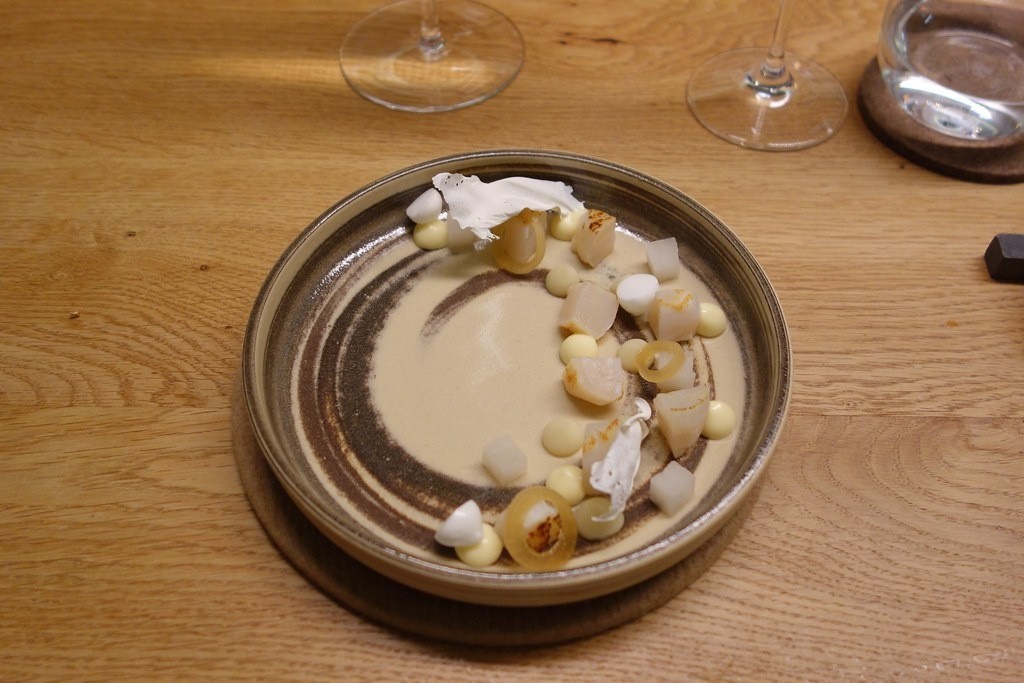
[341,1,525,111]
[687,1,849,149]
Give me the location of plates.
[239,150,791,607]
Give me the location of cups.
[875,1,1023,140]
